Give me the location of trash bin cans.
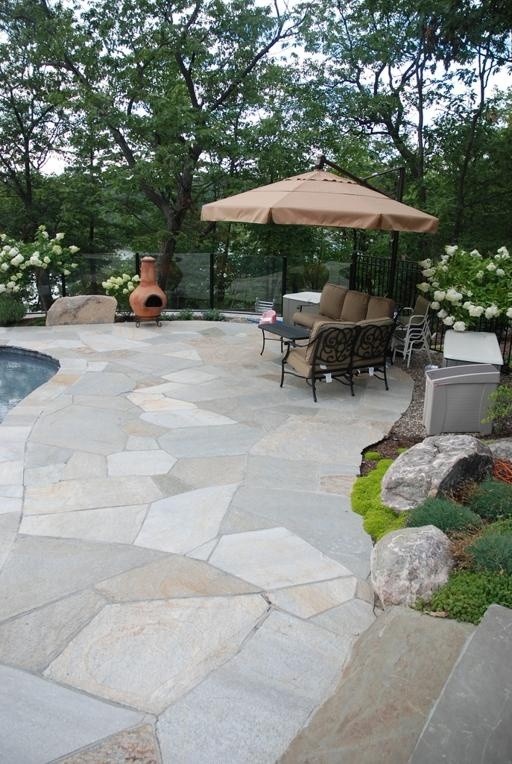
[423,364,500,434]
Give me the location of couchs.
[280,282,398,402]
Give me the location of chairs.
[392,295,433,368]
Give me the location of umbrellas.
[199,153,447,296]
